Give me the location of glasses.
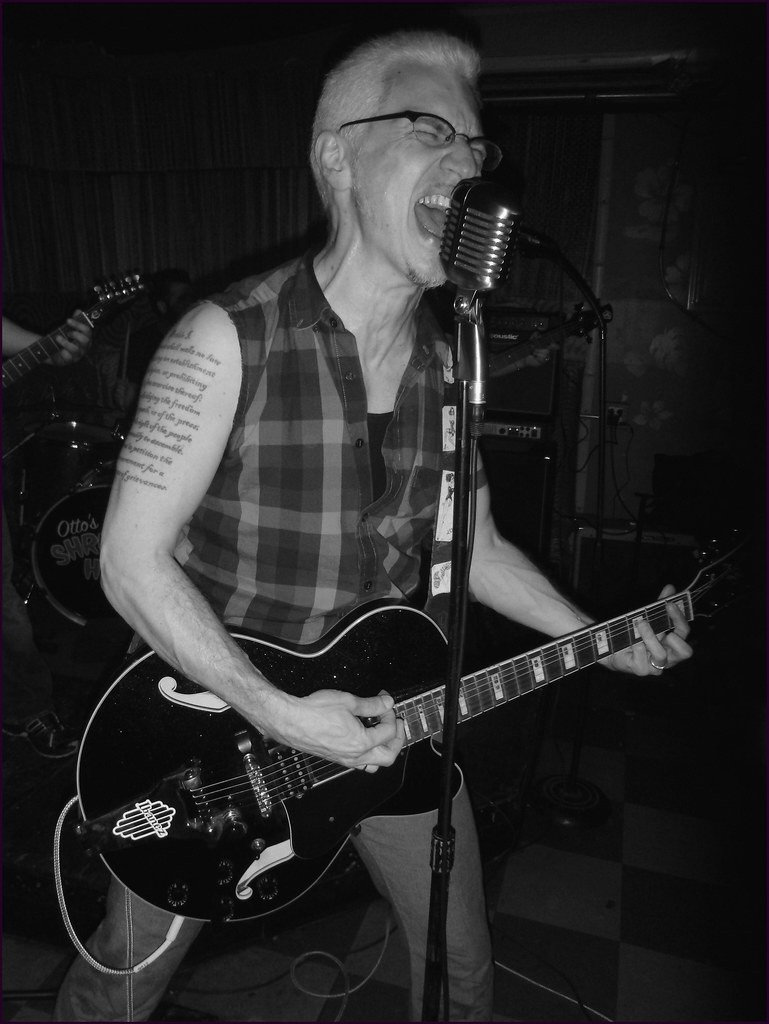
[337,108,504,173]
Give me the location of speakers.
[478,421,559,570]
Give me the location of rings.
[650,659,669,670]
[363,765,367,771]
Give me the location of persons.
[55,39,691,1023]
[1,310,93,759]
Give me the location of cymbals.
[0,397,119,415]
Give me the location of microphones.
[516,228,556,253]
[440,175,521,422]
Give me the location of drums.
[28,480,127,631]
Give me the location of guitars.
[72,511,769,926]
[0,266,161,396]
